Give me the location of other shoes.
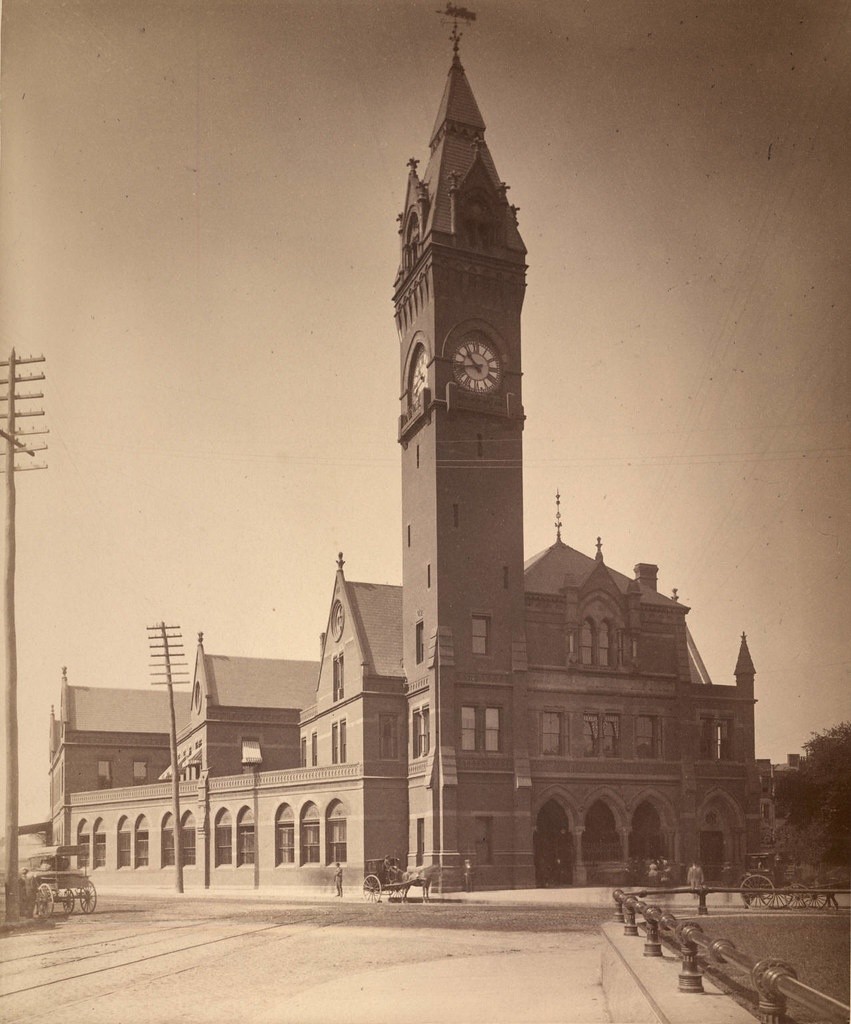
[335,895,340,897]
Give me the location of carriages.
[737,841,851,911]
[361,856,441,905]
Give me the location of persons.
[738,872,752,909]
[630,855,672,881]
[826,888,839,910]
[334,862,342,897]
[384,855,390,880]
[19,868,28,916]
[721,861,732,901]
[462,860,472,892]
[686,861,704,900]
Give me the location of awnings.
[241,740,263,764]
[181,746,202,768]
[158,755,186,779]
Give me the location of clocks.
[412,352,427,396]
[451,341,501,393]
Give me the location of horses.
[401,866,442,903]
